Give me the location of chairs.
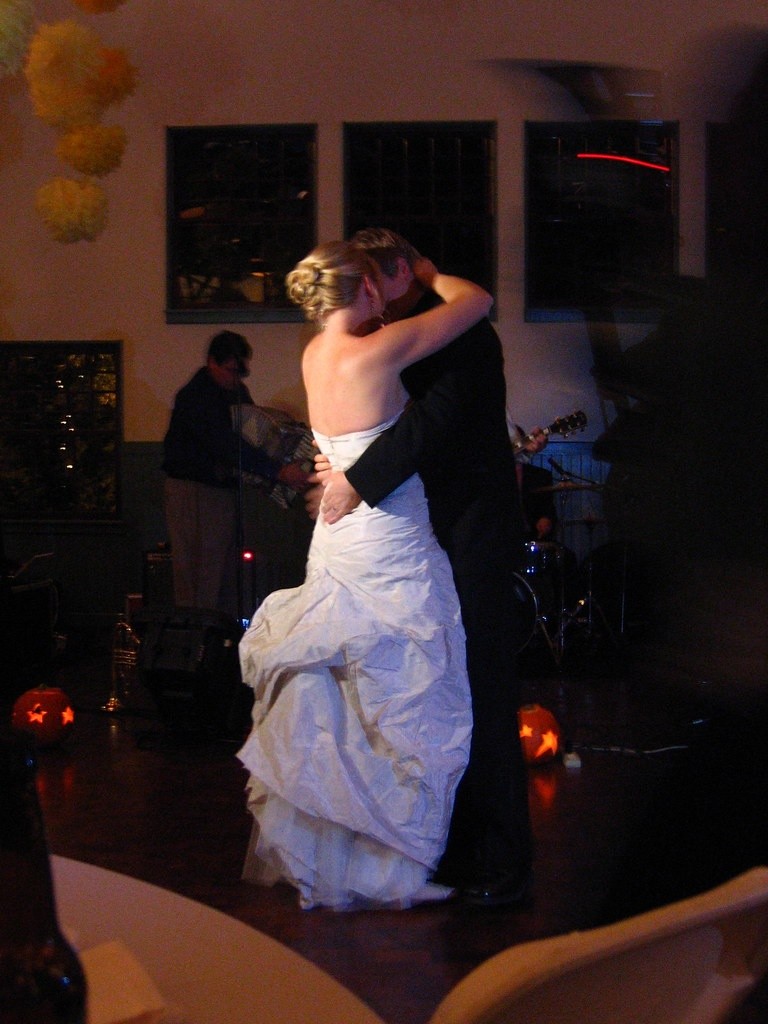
[430,867,768,1024]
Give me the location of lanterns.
[9,682,77,747]
[516,701,563,765]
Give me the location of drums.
[499,571,544,655]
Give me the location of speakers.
[128,607,255,741]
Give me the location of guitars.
[512,410,588,457]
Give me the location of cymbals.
[569,514,605,524]
[535,482,601,493]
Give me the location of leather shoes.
[464,868,533,904]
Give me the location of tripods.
[552,522,618,659]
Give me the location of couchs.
[161,457,313,617]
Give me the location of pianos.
[230,405,319,509]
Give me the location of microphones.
[547,458,570,481]
[232,345,248,378]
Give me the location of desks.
[47,853,391,1024]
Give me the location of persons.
[154,328,256,620]
[233,226,536,916]
